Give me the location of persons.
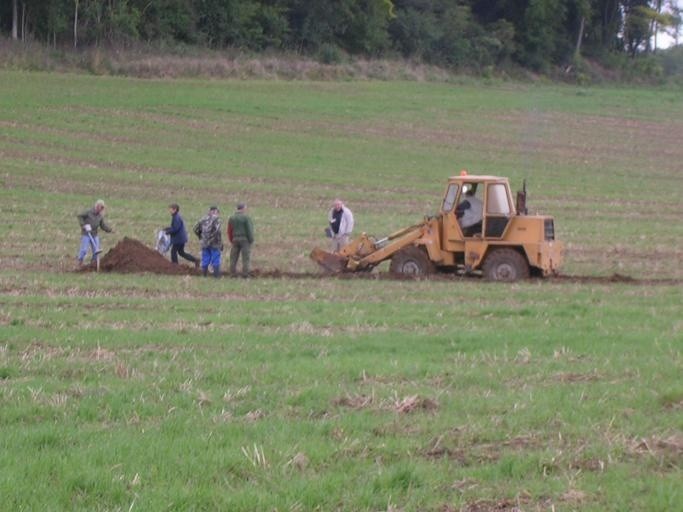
[455,189,484,235]
[161,201,200,273]
[76,199,116,269]
[227,201,254,278]
[327,197,354,250]
[193,205,225,278]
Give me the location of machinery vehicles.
[308,170,563,282]
